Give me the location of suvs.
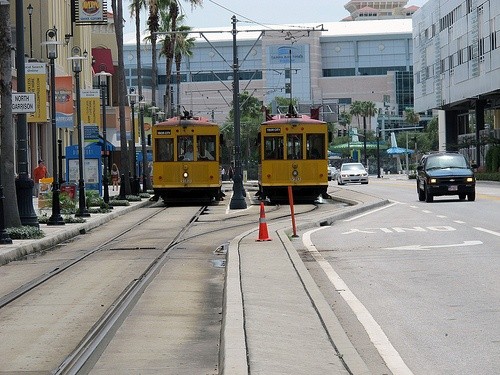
[415,150,478,203]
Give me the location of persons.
[34,160,47,189]
[184,145,194,161]
[111,164,119,191]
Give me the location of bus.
[257,49,334,202]
[147,105,225,203]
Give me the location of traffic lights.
[343,129,347,136]
[337,129,342,136]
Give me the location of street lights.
[26,3,34,58]
[148,101,159,127]
[125,86,139,196]
[136,95,147,193]
[67,46,91,217]
[39,28,66,226]
[94,63,114,210]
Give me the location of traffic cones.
[255,202,272,241]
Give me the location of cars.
[328,163,340,181]
[337,163,369,185]
